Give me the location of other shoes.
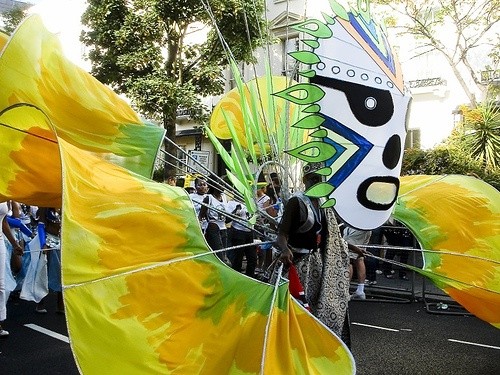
[0,329,9,335]
[36,307,47,313]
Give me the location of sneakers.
[351,291,366,301]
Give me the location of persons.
[380,218,413,280]
[0,167,285,337]
[363,227,382,286]
[276,161,351,351]
[342,225,372,301]
[374,227,395,275]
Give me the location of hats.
[301,161,323,176]
